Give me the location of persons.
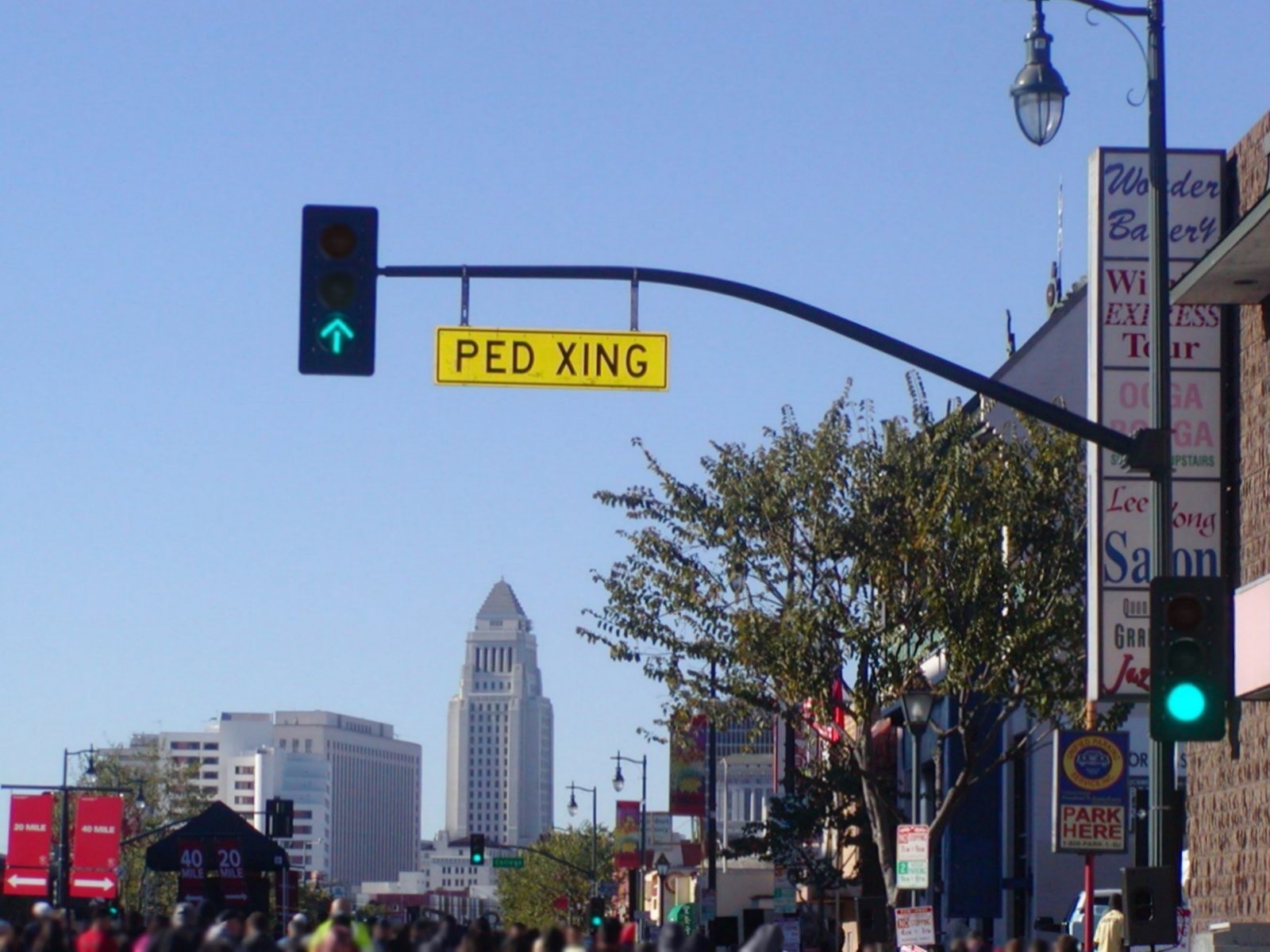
[0,893,1081,951]
[1092,892,1129,952]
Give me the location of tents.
[146,799,290,908]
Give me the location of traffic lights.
[590,897,604,934]
[300,203,378,375]
[1151,575,1232,741]
[468,833,487,866]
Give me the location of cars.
[1067,887,1122,945]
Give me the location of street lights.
[57,743,100,910]
[118,776,150,812]
[655,852,671,928]
[610,750,646,866]
[1010,2,1171,868]
[566,781,597,885]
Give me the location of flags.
[69,795,123,899]
[614,798,641,872]
[4,792,56,897]
[668,714,708,818]
[802,640,846,743]
